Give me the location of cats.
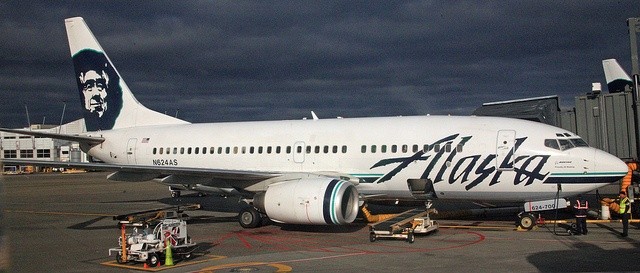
[1,15,628,242]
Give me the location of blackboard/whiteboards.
[107,219,203,267]
[370,214,439,244]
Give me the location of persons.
[618,191,631,237]
[79,69,110,119]
[573,194,588,235]
